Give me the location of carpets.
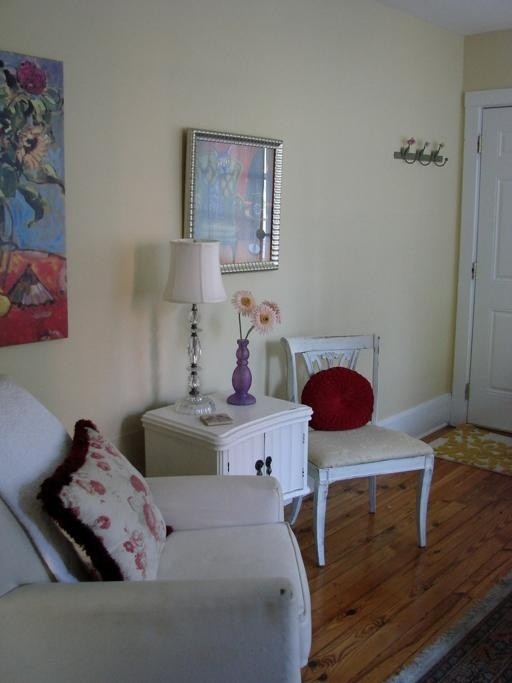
[426,421,511,480]
[384,566,512,682]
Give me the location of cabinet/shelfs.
[141,389,313,527]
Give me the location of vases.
[227,339,256,406]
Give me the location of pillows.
[300,365,376,433]
[39,419,174,582]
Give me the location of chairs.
[279,333,435,569]
[0,371,314,683]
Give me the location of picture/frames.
[184,127,284,274]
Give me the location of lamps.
[160,235,229,413]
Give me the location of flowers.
[229,288,284,342]
